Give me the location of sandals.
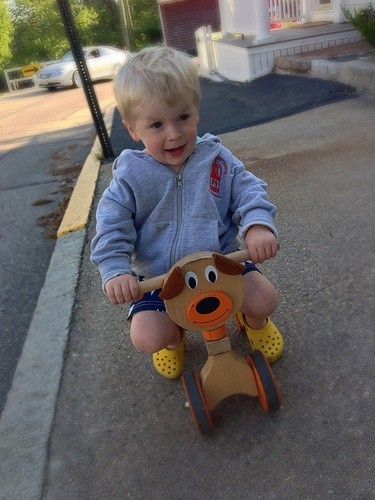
[236,310,285,363]
[151,324,186,379]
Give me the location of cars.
[34,45,132,92]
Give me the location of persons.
[89,46,285,379]
